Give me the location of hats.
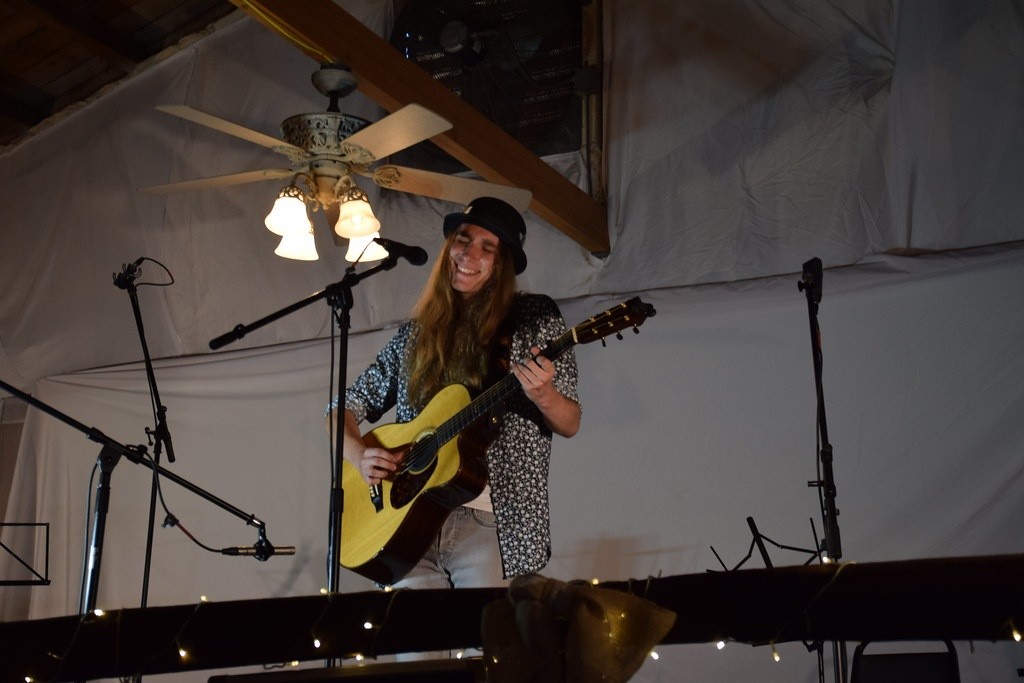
[443,196,527,275]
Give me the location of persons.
[323,194,582,594]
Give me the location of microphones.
[800,257,824,307]
[114,257,145,288]
[373,238,428,265]
[222,540,295,562]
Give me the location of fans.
[130,64,531,218]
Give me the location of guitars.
[338,296,657,586]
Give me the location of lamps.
[333,175,382,238]
[265,171,318,235]
[343,231,389,263]
[273,197,321,262]
